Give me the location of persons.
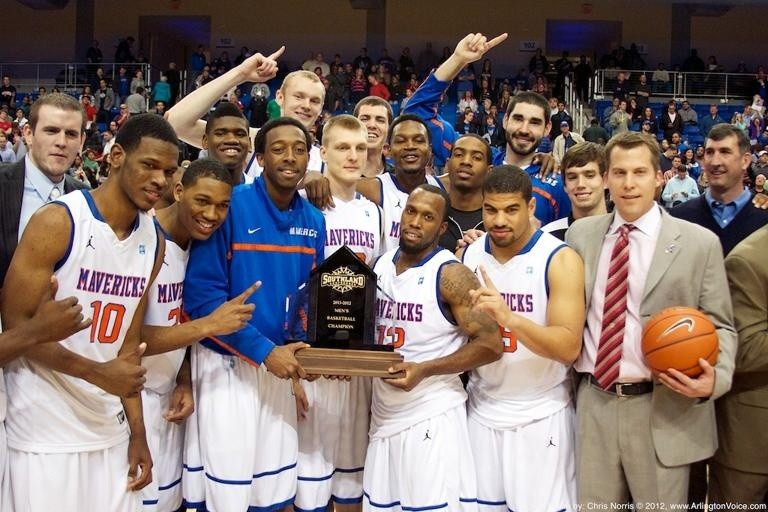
[0,31,767,512]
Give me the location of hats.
[560,121,568,126]
[758,150,767,157]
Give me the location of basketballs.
[641,306,719,378]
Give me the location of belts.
[584,372,655,396]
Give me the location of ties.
[595,225,638,388]
